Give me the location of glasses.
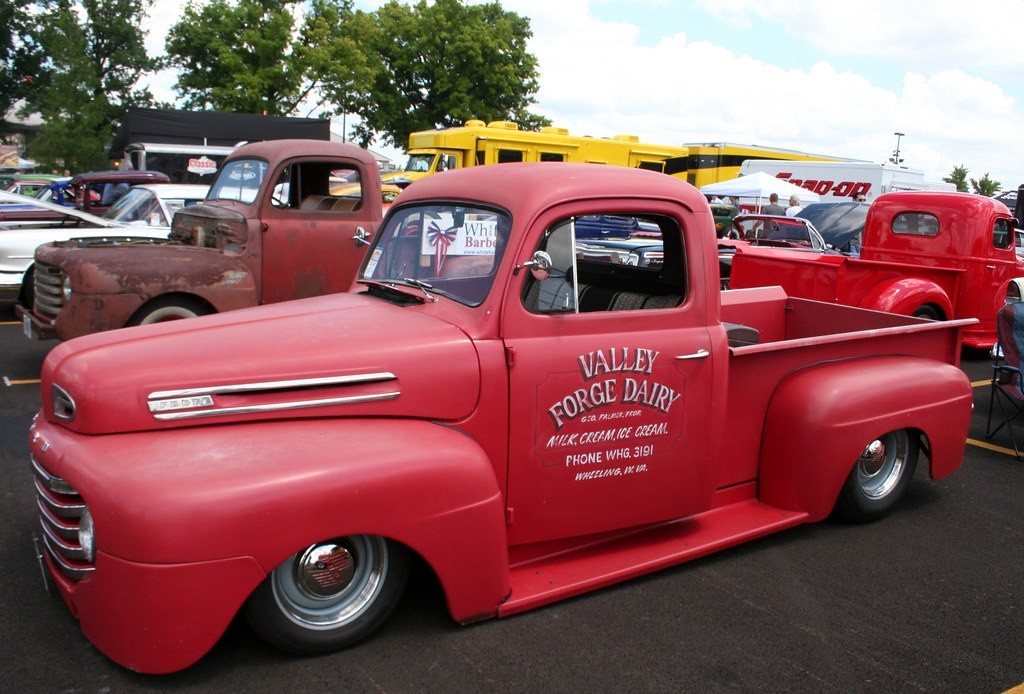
[857,199,867,203]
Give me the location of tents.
[110,104,330,146]
[700,171,819,238]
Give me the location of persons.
[729,196,739,209]
[751,193,786,238]
[51,169,59,175]
[710,195,723,203]
[64,169,69,176]
[101,167,131,207]
[786,194,804,217]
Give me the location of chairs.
[524,279,601,311]
[607,288,685,311]
[983,301,1024,462]
[330,198,363,211]
[300,194,338,210]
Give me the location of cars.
[0,138,497,343]
[571,201,877,291]
[1012,228,1024,257]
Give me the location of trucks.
[324,117,879,201]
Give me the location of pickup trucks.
[27,159,982,676]
[723,189,1024,353]
